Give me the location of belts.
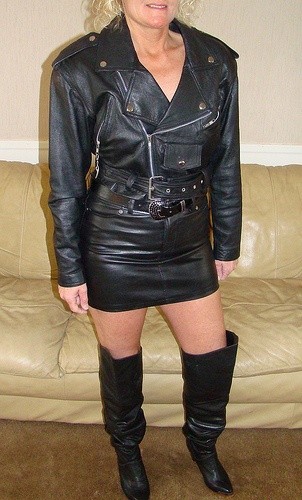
[94,183,193,220]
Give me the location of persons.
[49,0,242,500]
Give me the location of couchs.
[0,160,302,430]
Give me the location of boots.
[97,344,150,500]
[180,331,239,496]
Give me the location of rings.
[234,265,237,268]
